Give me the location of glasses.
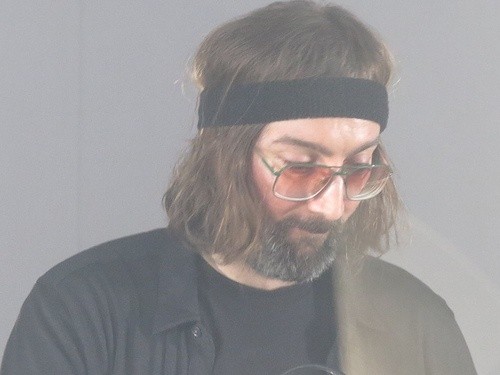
[251,144,391,201]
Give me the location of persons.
[0,0,478,375]
